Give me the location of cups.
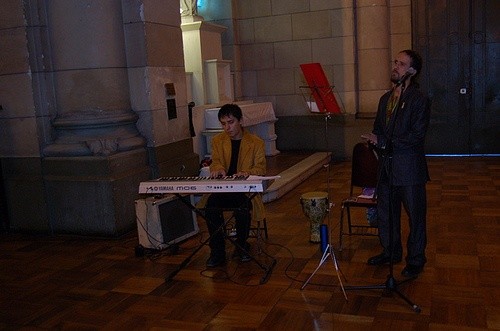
[320,224,328,253]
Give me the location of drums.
[300,191,331,244]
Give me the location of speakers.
[135,194,200,250]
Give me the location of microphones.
[398,67,414,83]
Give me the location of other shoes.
[206,253,226,266]
[368,253,402,263]
[238,245,252,261]
[401,265,424,274]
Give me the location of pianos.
[134,175,278,286]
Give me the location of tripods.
[299,62,349,289]
[339,80,421,314]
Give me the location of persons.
[361,49,431,277]
[205,104,267,268]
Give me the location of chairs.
[227,210,269,254]
[338,141,385,247]
